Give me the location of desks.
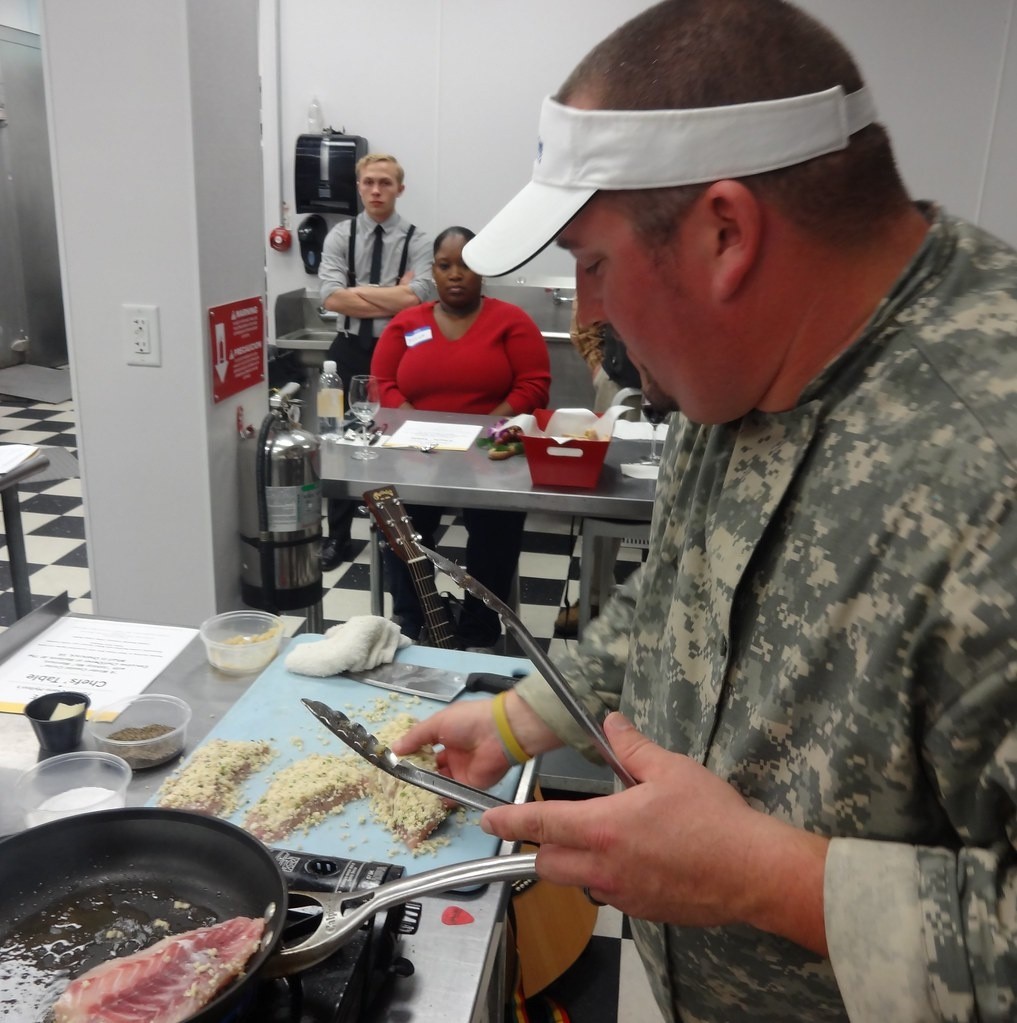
[0,455,51,619]
[321,402,665,617]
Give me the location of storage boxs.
[517,408,614,488]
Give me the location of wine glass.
[641,392,668,466]
[349,375,381,460]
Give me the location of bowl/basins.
[87,695,193,768]
[10,752,133,830]
[200,610,286,674]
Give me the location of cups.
[23,691,91,752]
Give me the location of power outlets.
[122,304,161,366]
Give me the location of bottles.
[306,93,322,135]
[316,361,343,441]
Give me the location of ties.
[370,225,384,285]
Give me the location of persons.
[555,295,664,635]
[317,155,435,570]
[371,226,551,653]
[392,0,1017,1022]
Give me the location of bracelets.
[493,691,532,768]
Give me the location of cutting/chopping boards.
[143,632,540,896]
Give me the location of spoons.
[383,445,438,452]
[361,427,381,440]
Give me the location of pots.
[0,806,539,1023]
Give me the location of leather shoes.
[318,535,353,571]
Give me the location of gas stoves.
[189,848,423,1023]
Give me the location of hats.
[458,82,877,278]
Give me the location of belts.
[335,330,379,346]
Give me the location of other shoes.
[464,646,496,655]
[388,611,424,629]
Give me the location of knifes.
[339,661,521,703]
[369,423,387,446]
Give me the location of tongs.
[298,542,639,812]
